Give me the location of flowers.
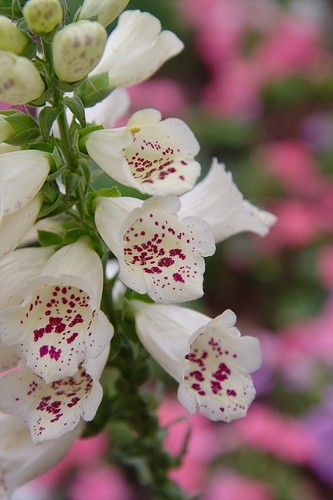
[0,1,332,499]
